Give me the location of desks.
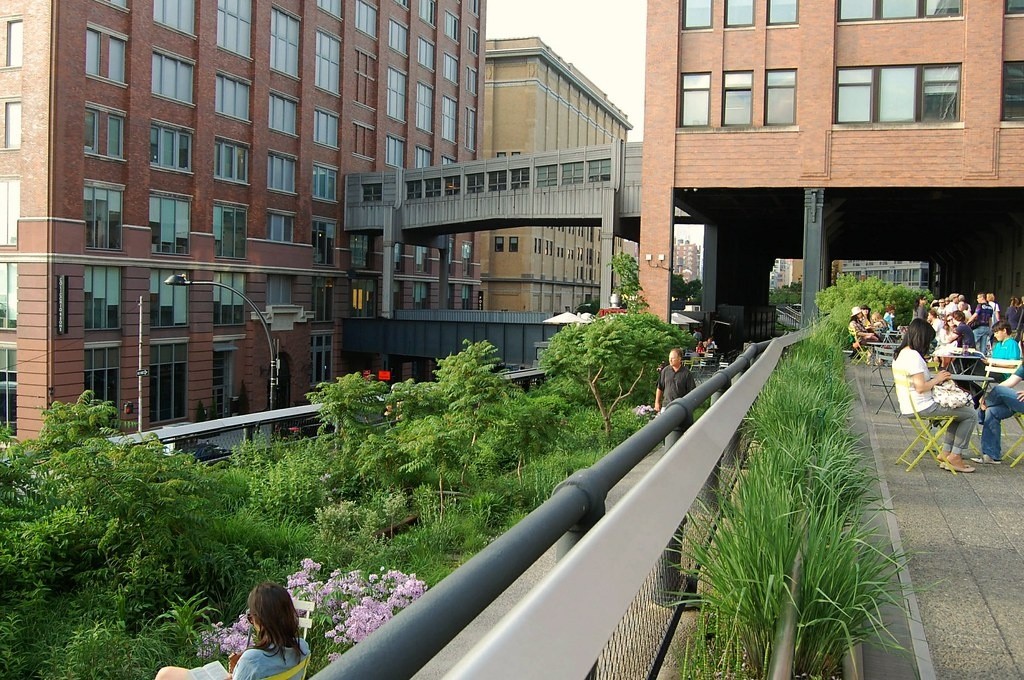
[929,374,994,467]
[867,342,902,387]
[881,333,902,343]
[929,353,981,376]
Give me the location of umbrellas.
[542,311,586,327]
[671,313,701,324]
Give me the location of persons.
[679,324,714,370]
[154,582,311,680]
[970,330,1024,464]
[848,292,1024,409]
[891,317,977,472]
[654,348,696,445]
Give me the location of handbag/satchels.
[1010,330,1017,340]
[932,379,967,409]
[969,304,982,330]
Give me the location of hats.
[861,306,871,312]
[850,306,863,317]
[949,293,959,301]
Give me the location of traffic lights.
[124,402,132,414]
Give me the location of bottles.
[962,342,968,356]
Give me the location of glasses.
[246,613,256,624]
[947,318,953,322]
[922,297,927,300]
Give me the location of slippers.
[970,453,1002,465]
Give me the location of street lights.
[163,274,277,411]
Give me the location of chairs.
[256,652,311,680]
[246,600,315,648]
[892,368,957,476]
[847,326,873,366]
[875,346,897,415]
[975,358,1023,442]
[1001,412,1024,468]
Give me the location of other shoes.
[944,461,975,473]
[940,461,971,469]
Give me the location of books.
[189,660,229,680]
